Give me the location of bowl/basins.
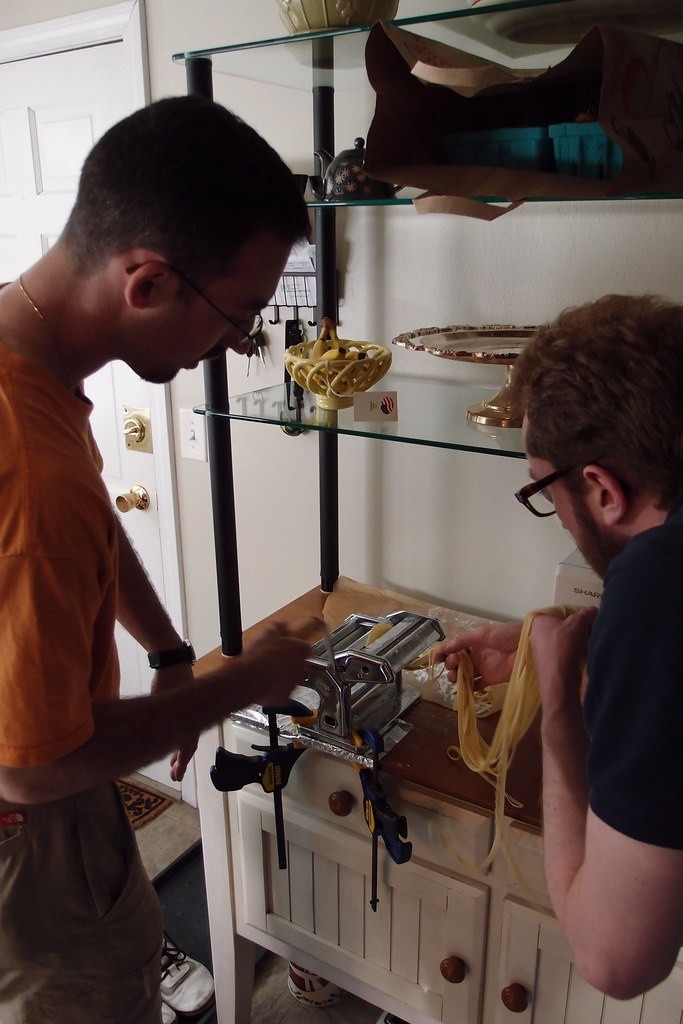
[273,0,399,36]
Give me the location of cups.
[293,174,308,197]
[308,176,326,201]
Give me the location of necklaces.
[20,275,74,391]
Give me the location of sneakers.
[156,941,219,1024]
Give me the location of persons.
[431,295,682,1002]
[0,97,312,1024]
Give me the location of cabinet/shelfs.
[171,0,683,660]
[192,584,683,1024]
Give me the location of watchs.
[148,639,196,669]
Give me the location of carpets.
[116,776,173,832]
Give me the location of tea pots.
[313,137,408,201]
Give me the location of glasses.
[123,260,264,344]
[513,459,577,518]
